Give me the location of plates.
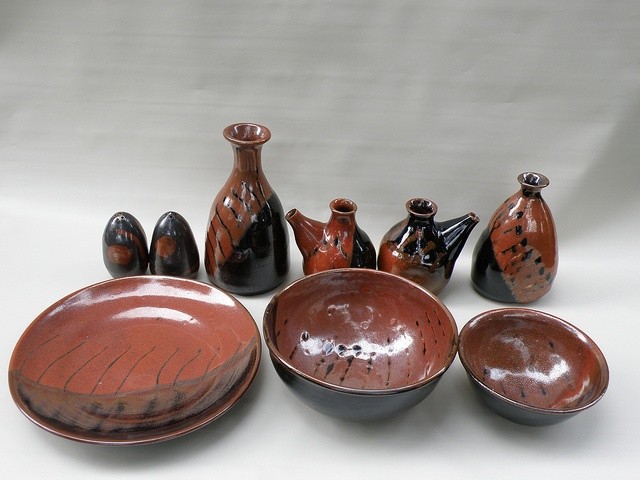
[7,275,261,446]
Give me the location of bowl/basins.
[458,308,609,425]
[262,268,458,421]
